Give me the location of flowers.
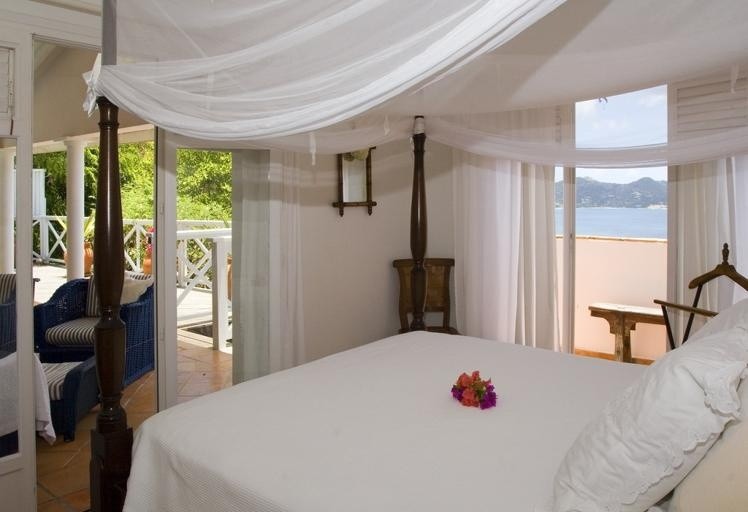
[137,225,155,255]
[451,372,496,409]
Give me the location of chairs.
[393,259,460,336]
[18,278,153,442]
[0,274,39,357]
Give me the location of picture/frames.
[332,145,376,216]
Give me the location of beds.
[121,328,748,512]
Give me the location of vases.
[143,253,152,274]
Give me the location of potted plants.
[54,208,97,277]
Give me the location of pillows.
[552,298,748,512]
[85,275,153,318]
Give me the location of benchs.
[588,302,666,365]
